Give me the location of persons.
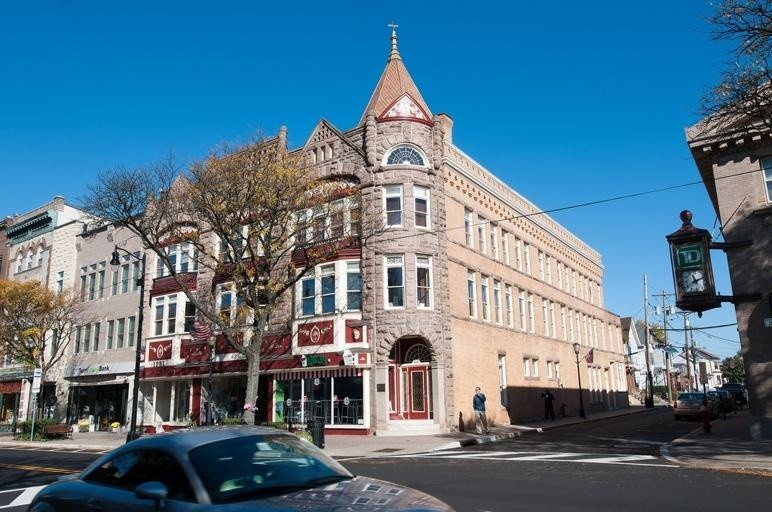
[200,400,214,425]
[540,388,556,421]
[472,386,489,435]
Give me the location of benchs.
[41,423,74,439]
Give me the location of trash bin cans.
[307,418,325,448]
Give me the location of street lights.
[572,342,583,408]
[109,246,147,434]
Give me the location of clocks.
[663,209,764,317]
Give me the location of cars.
[672,383,748,421]
[27,427,454,512]
